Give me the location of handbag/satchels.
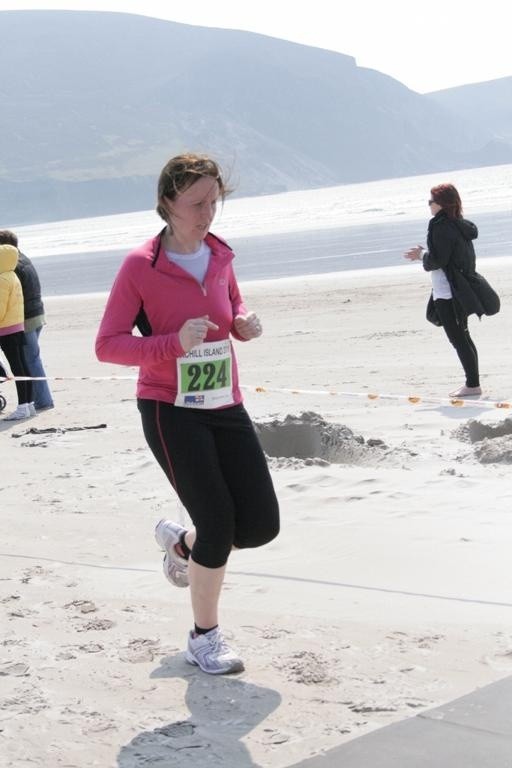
[426,290,443,326]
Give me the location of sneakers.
[3,403,52,420]
[186,628,244,674]
[449,384,481,398]
[154,519,190,587]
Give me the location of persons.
[95,154,280,674]
[0,230,54,411]
[403,185,499,398]
[0,244,36,421]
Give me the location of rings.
[255,325,261,332]
